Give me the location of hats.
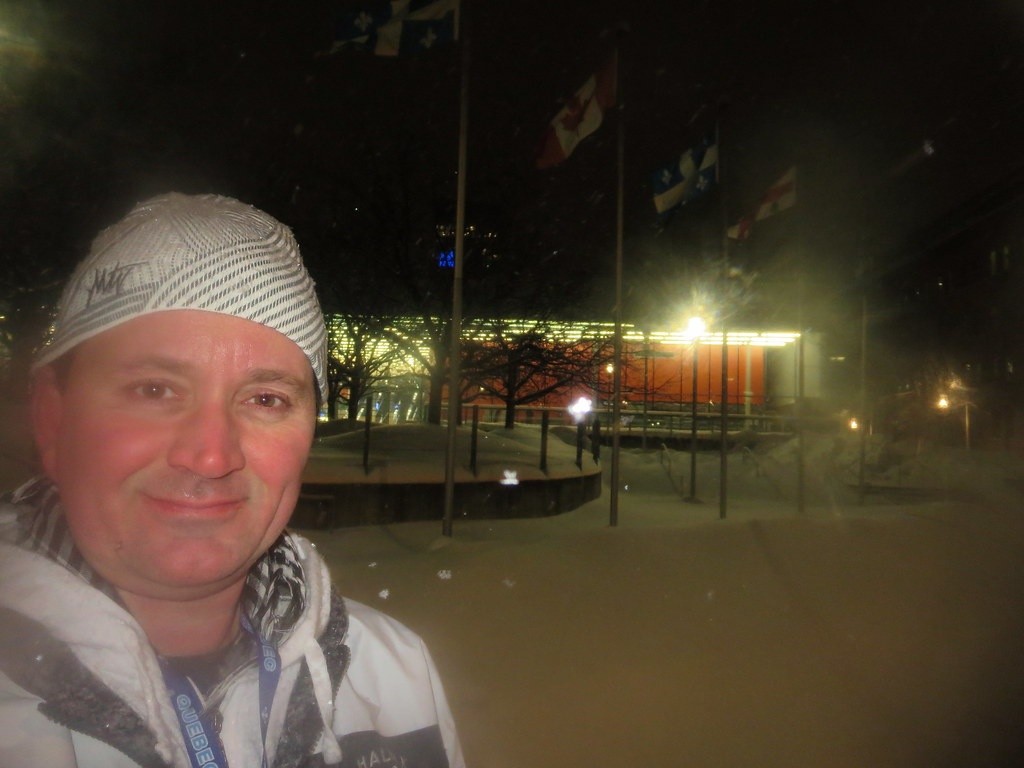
[32,195,329,404]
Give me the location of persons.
[1,190,463,768]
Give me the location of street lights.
[607,364,615,448]
[680,315,705,504]
